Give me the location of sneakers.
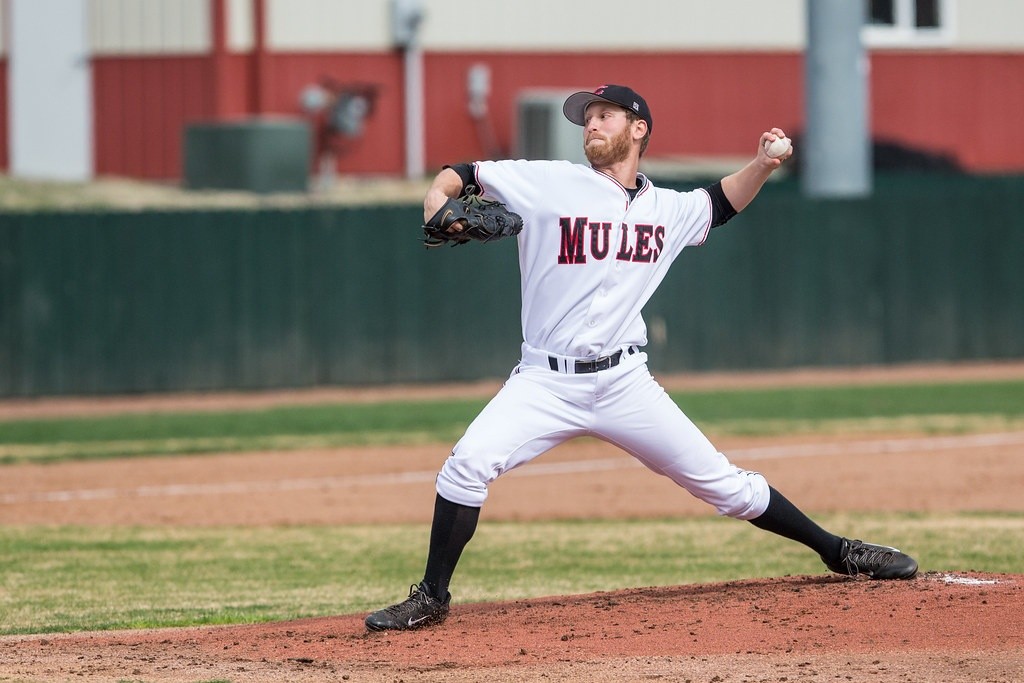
[820,537,919,579]
[364,581,452,633]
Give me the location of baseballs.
[764,134,790,158]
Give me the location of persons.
[364,84,917,633]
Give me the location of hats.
[563,84,653,135]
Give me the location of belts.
[547,344,634,374]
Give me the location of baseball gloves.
[423,194,524,243]
